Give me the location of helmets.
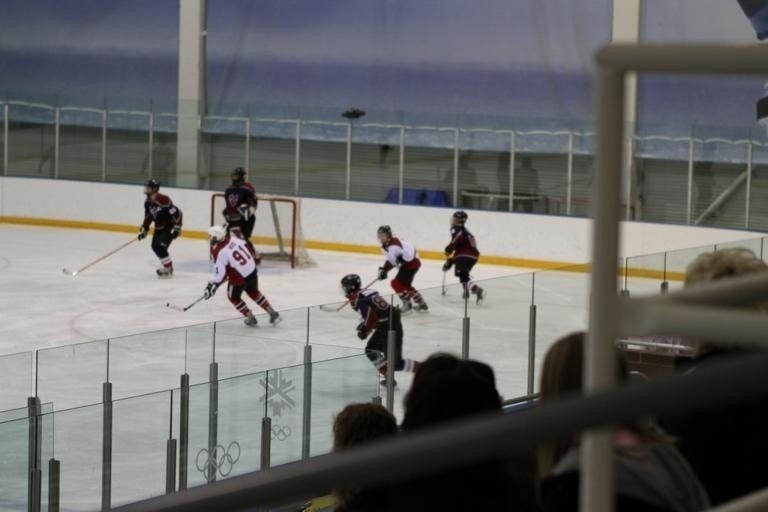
[232,168,244,183]
[341,274,360,293]
[144,178,160,194]
[377,226,391,238]
[453,210,467,224]
[208,226,225,242]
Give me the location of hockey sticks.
[442,254,450,295]
[319,276,380,311]
[63,237,137,276]
[166,284,220,312]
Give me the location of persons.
[203,225,280,326]
[138,177,182,274]
[210,166,261,263]
[377,225,430,311]
[442,210,484,300]
[304,248,768,511]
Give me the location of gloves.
[445,247,452,257]
[442,261,451,271]
[358,326,367,338]
[378,267,387,280]
[204,283,218,299]
[138,228,147,239]
[170,227,179,237]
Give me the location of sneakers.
[412,303,427,310]
[244,316,257,324]
[476,287,483,304]
[156,268,172,275]
[270,312,278,322]
[400,303,411,312]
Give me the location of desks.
[384,185,635,229]
